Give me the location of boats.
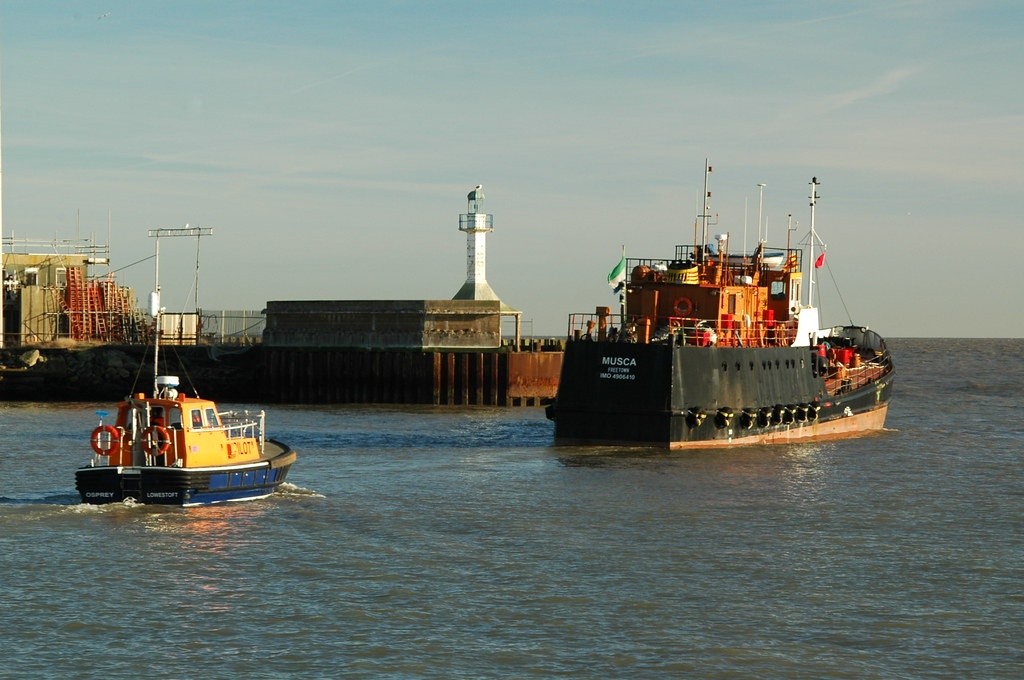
[74,283,300,503]
[546,157,896,451]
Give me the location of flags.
[814,253,826,268]
[606,256,626,295]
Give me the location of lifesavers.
[673,296,692,317]
[740,400,821,430]
[689,407,707,427]
[718,407,734,426]
[140,425,172,457]
[90,425,120,456]
